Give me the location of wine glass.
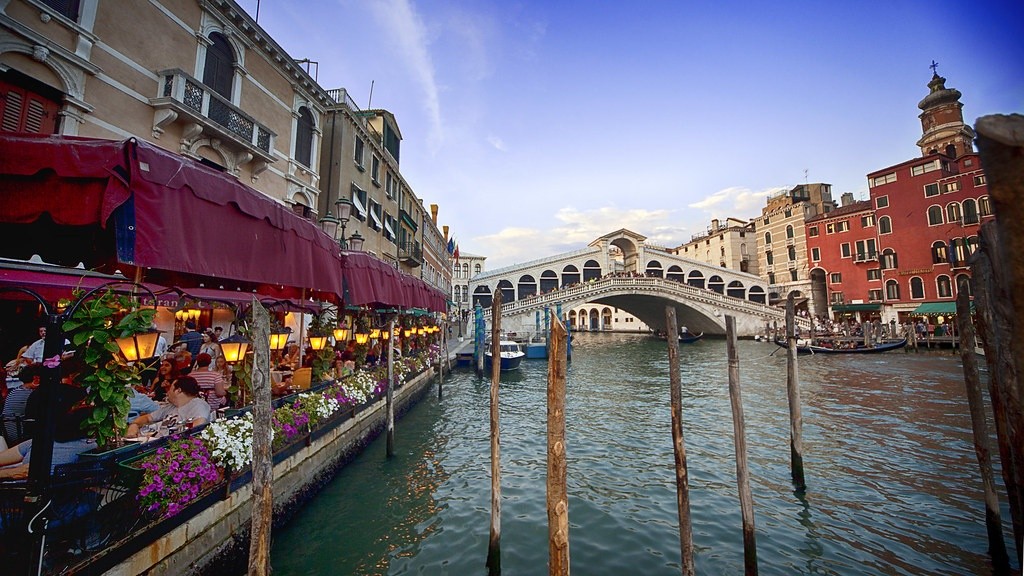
[185,416,198,430]
[140,423,158,445]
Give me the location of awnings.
[910,301,974,316]
[833,304,884,312]
[776,298,808,308]
[0,128,447,368]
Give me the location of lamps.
[68,280,439,361]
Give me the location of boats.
[654,329,703,343]
[773,331,908,354]
[484,342,525,372]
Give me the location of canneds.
[168,427,178,435]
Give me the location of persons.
[0,318,441,530]
[527,268,714,298]
[794,312,952,350]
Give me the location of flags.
[448,237,459,265]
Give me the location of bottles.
[274,358,278,371]
[177,422,184,432]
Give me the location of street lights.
[321,194,365,352]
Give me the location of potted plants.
[61,290,140,475]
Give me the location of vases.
[76,373,356,492]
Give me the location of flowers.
[135,342,446,517]
